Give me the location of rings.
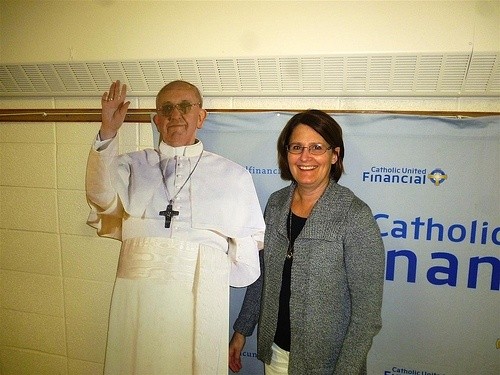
[102,95,108,100]
[108,96,113,102]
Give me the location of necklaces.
[286,189,325,258]
[156,150,204,228]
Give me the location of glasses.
[286,142,332,155]
[155,101,201,118]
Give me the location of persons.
[84,78,265,374]
[228,109,385,375]
